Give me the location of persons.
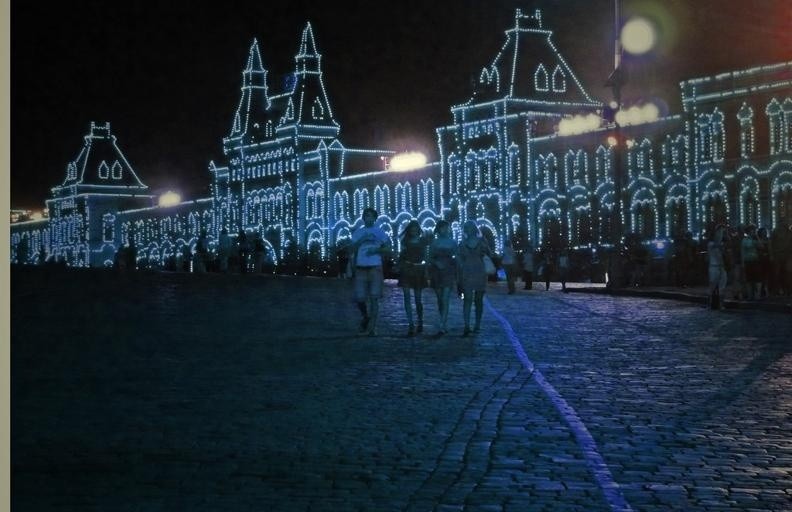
[348,207,392,337]
[672,216,792,310]
[499,234,565,292]
[396,218,499,338]
[137,227,353,279]
[11,233,87,266]
[619,229,651,288]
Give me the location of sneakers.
[357,318,481,336]
